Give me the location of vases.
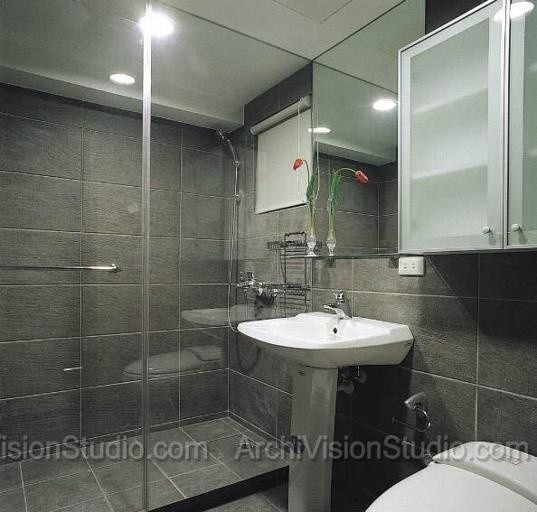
[306,228,316,255]
[326,231,337,256]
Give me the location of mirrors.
[301,0,425,256]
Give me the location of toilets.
[121,344,223,381]
[364,438,537,511]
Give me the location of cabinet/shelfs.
[268,232,311,318]
[398,0,537,255]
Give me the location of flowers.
[294,158,319,236]
[328,168,369,233]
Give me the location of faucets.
[321,292,352,319]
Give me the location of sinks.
[179,303,296,337]
[236,312,414,369]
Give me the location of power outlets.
[397,256,424,276]
